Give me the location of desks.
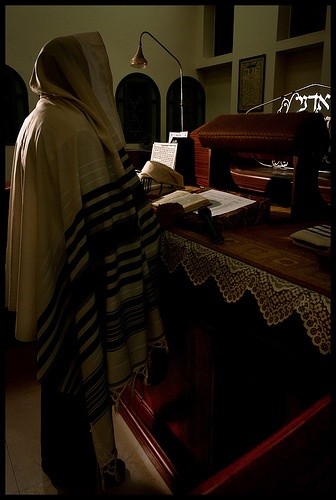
[104,187,335,500]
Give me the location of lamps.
[129,31,185,132]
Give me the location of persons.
[5,31,169,494]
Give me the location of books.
[151,189,213,214]
